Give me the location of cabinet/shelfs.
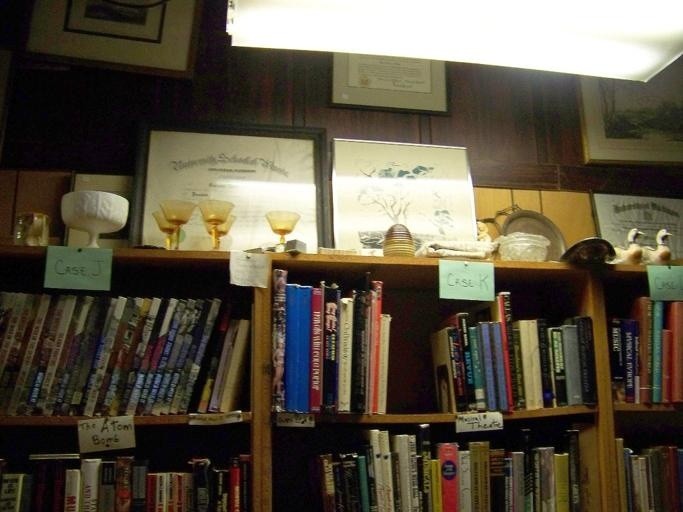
[597,246,681,508]
[0,246,261,512]
[262,244,611,508]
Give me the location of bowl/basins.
[499,231,551,262]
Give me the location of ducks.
[614,227,645,267]
[644,229,673,265]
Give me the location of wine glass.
[58,190,129,247]
[199,198,236,249]
[152,210,175,250]
[264,209,300,244]
[159,200,198,248]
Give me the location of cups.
[12,210,52,247]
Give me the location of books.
[430,292,598,415]
[615,437,683,512]
[312,430,582,512]
[606,297,683,405]
[0,292,250,420]
[0,454,252,512]
[271,269,392,415]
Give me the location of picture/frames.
[59,169,136,247]
[589,190,683,265]
[327,52,454,117]
[573,74,683,168]
[127,117,333,252]
[14,0,206,80]
[333,137,478,254]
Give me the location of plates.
[501,210,566,261]
[560,237,615,266]
